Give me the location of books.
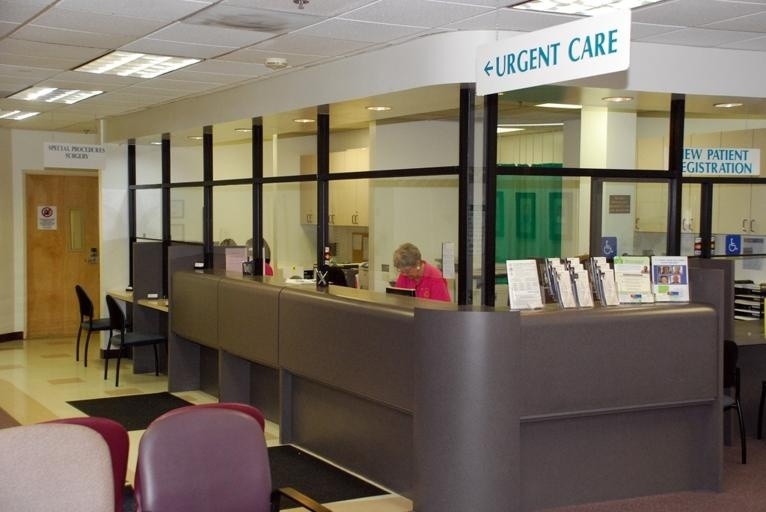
[545,256,620,309]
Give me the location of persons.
[674,266,682,274]
[392,242,450,302]
[659,275,669,283]
[661,266,673,274]
[670,275,681,284]
[640,265,649,274]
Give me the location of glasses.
[397,263,417,275]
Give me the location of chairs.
[75,285,128,367]
[133,405,266,510]
[138,411,329,512]
[0,422,138,512]
[39,418,130,512]
[723,340,747,464]
[104,294,160,387]
[756,380,766,440]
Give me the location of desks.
[734,320,766,346]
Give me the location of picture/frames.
[496,191,573,243]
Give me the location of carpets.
[66,391,194,431]
[267,445,392,509]
